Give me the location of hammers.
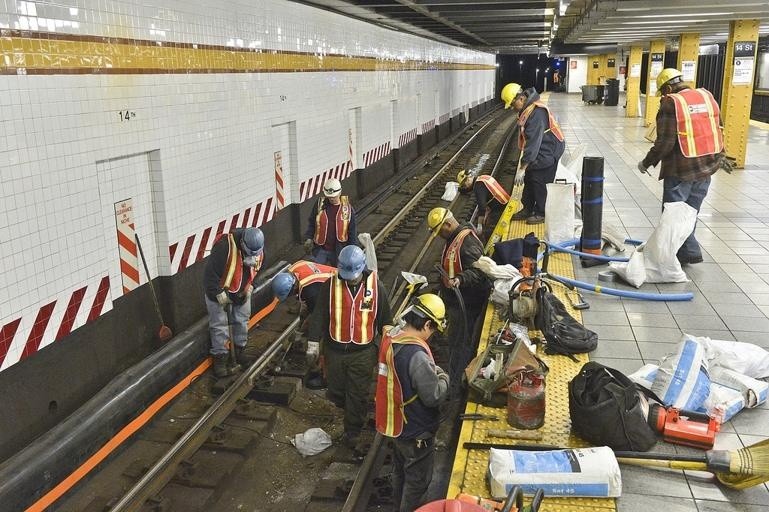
[227,300,242,374]
[270,342,293,375]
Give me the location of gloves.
[476,223,484,237]
[214,291,230,305]
[513,169,527,188]
[637,159,650,175]
[304,341,324,369]
[303,239,315,256]
[247,284,255,297]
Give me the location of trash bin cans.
[604,78,619,106]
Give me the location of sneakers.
[212,354,229,379]
[343,426,365,451]
[234,345,254,366]
[512,207,535,221]
[305,374,328,390]
[526,212,545,225]
[309,365,323,378]
[676,252,705,267]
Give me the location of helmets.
[240,225,266,257]
[500,82,522,110]
[654,67,685,98]
[271,271,295,303]
[456,169,472,188]
[336,244,367,281]
[412,292,447,334]
[426,205,451,239]
[322,178,343,198]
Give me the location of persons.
[425,207,491,420]
[272,259,338,333]
[499,83,566,222]
[203,226,265,378]
[457,169,510,237]
[374,292,453,511]
[303,178,356,268]
[637,68,724,265]
[308,249,393,447]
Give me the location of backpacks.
[533,284,600,355]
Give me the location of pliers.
[459,413,502,420]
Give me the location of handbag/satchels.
[567,360,660,452]
[460,329,551,411]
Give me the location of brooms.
[463,441,769,477]
